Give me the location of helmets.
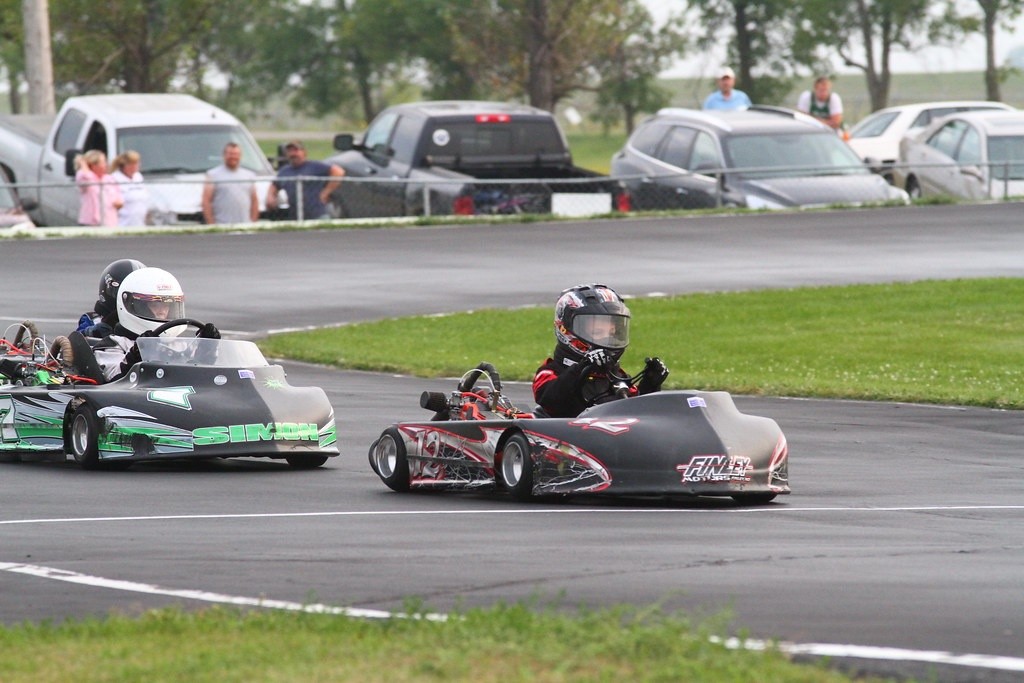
[95,259,147,320]
[118,265,187,342]
[554,283,631,371]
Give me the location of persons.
[73,149,123,228]
[702,66,755,109]
[110,149,147,226]
[75,258,148,339]
[91,266,221,384]
[266,138,345,220]
[201,142,259,224]
[532,283,669,417]
[798,77,844,127]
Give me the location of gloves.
[197,323,222,350]
[577,346,611,373]
[637,355,669,394]
[86,322,113,339]
[125,328,162,368]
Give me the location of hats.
[285,140,305,150]
[715,66,735,76]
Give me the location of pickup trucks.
[320,100,631,219]
[0,93,289,228]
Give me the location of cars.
[843,102,1024,202]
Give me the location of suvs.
[611,104,911,209]
